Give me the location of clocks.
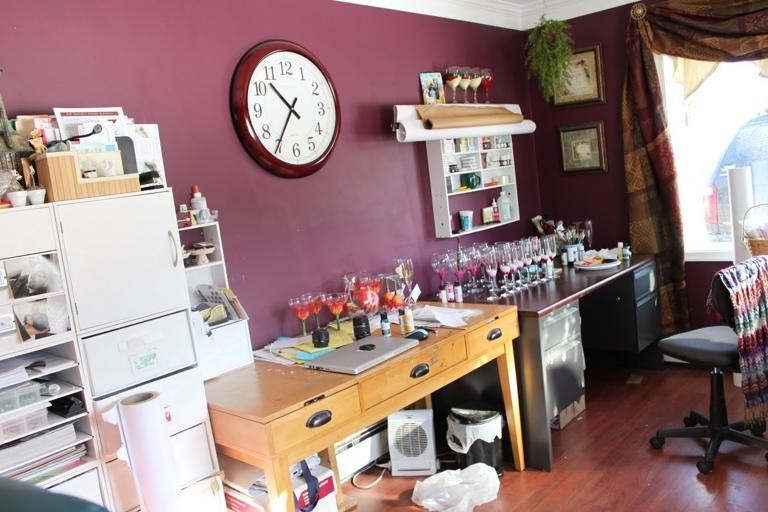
[231,38,342,180]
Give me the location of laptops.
[304,334,420,376]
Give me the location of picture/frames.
[547,43,606,111]
[558,120,608,177]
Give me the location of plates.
[572,258,621,270]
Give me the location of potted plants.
[522,13,578,103]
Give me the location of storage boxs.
[217,453,337,512]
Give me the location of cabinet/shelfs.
[426,135,522,239]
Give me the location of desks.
[422,256,657,473]
[205,301,520,512]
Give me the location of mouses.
[405,328,429,340]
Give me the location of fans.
[386,409,441,477]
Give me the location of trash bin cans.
[450,407,504,477]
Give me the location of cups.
[6,190,27,207]
[311,329,329,348]
[457,210,474,232]
[27,189,45,205]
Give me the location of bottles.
[491,199,502,223]
[560,249,569,266]
[378,313,394,337]
[352,315,371,341]
[618,241,631,260]
[191,185,208,222]
[497,191,513,221]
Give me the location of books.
[0,350,88,487]
[192,282,251,322]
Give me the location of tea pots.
[460,173,480,190]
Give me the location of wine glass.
[584,218,593,250]
[286,259,413,344]
[443,66,494,104]
[430,236,560,305]
[188,247,215,265]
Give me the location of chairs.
[647,255,768,477]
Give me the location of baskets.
[740,204,767,257]
